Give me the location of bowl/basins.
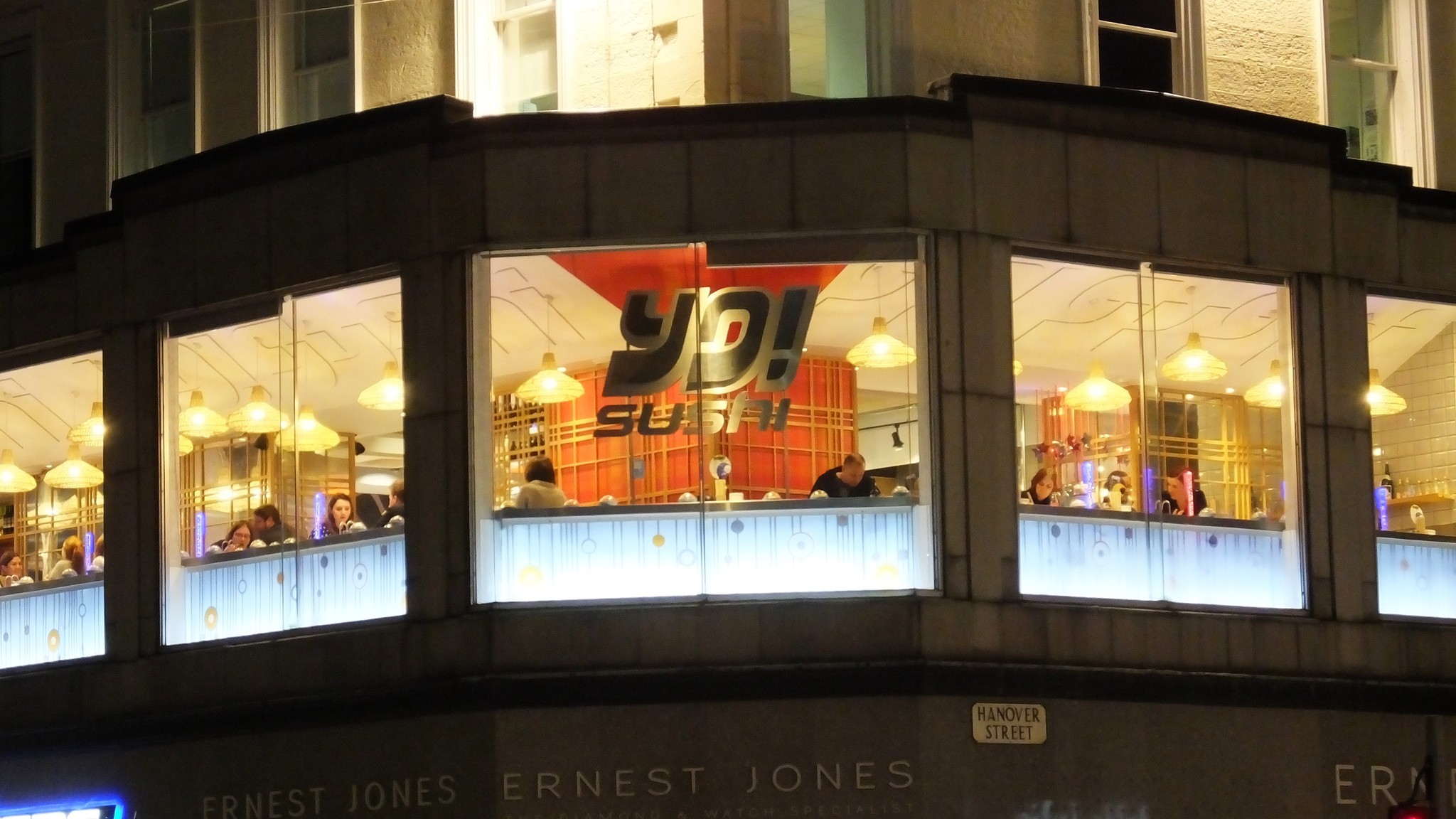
[1199,513,1216,517]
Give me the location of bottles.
[1049,492,1059,506]
[1378,459,1449,500]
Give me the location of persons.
[208,519,255,553]
[808,451,880,498]
[48,536,86,579]
[0,551,26,588]
[517,456,567,508]
[1158,464,1209,517]
[1093,470,1137,511]
[1021,468,1061,506]
[93,534,104,572]
[253,504,306,546]
[306,493,354,540]
[373,479,404,530]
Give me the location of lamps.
[846,266,916,367]
[1163,286,1228,382]
[225,336,290,434]
[1368,313,1407,415]
[1064,358,1132,411]
[0,394,37,492]
[275,320,340,452]
[43,391,103,489]
[178,343,227,438]
[1244,310,1282,409]
[357,311,404,410]
[66,360,103,447]
[514,294,585,404]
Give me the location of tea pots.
[1251,507,1268,518]
